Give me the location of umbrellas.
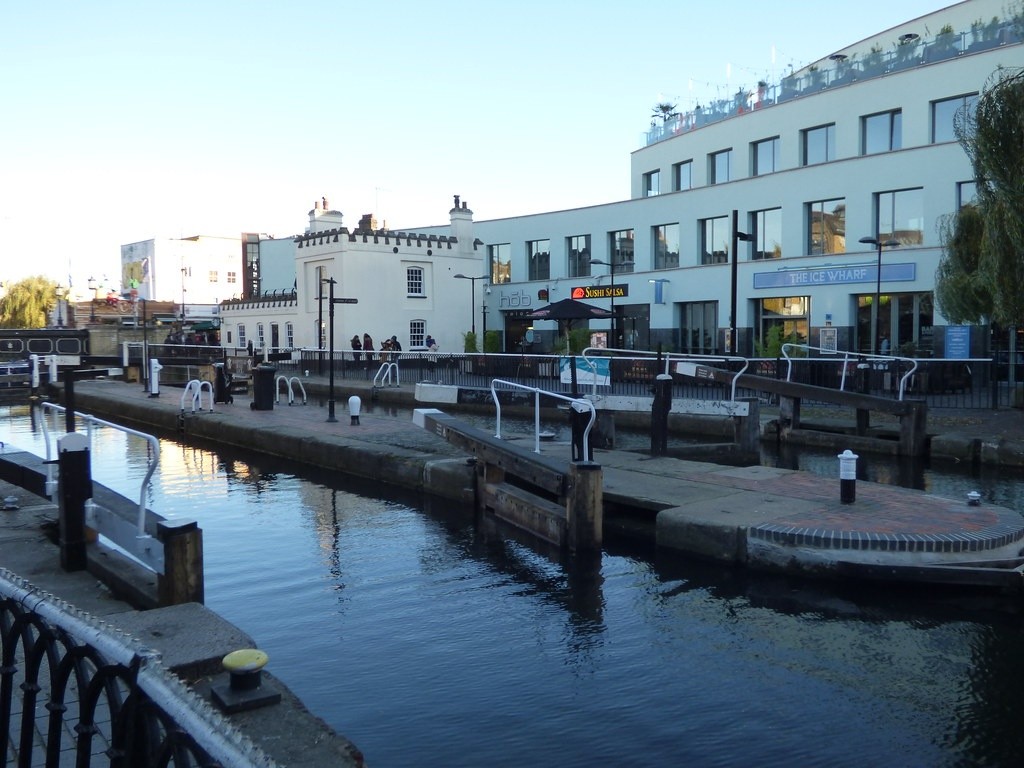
[515,298,627,356]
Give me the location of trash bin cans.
[212,363,234,404]
[250,366,276,411]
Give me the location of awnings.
[190,324,220,330]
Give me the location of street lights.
[181,268,188,325]
[858,235,903,361]
[133,296,154,393]
[87,276,99,323]
[587,258,636,357]
[320,277,340,423]
[453,273,491,335]
[54,284,66,329]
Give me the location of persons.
[426,335,439,372]
[379,335,402,377]
[164,332,221,358]
[351,335,362,369]
[363,333,375,369]
[880,335,890,355]
